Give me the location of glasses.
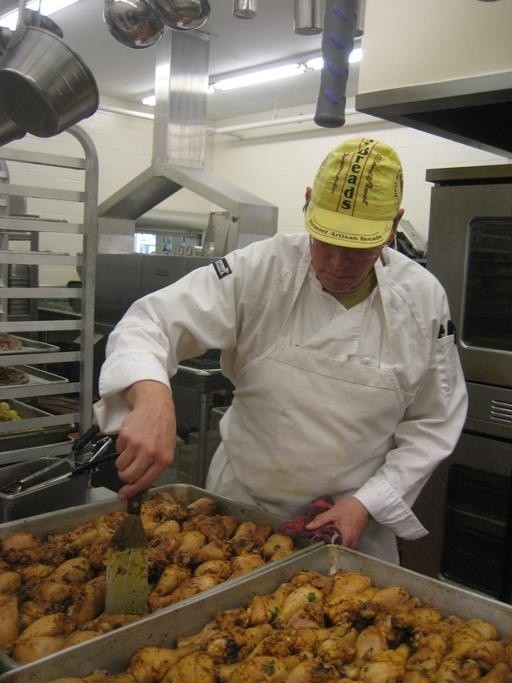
[311,237,393,262]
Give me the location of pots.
[1,0,100,139]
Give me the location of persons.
[90,134,473,554]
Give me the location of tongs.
[2,434,113,499]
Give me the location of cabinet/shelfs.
[0,118,103,519]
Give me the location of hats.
[303,136,404,250]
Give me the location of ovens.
[396,163,512,605]
